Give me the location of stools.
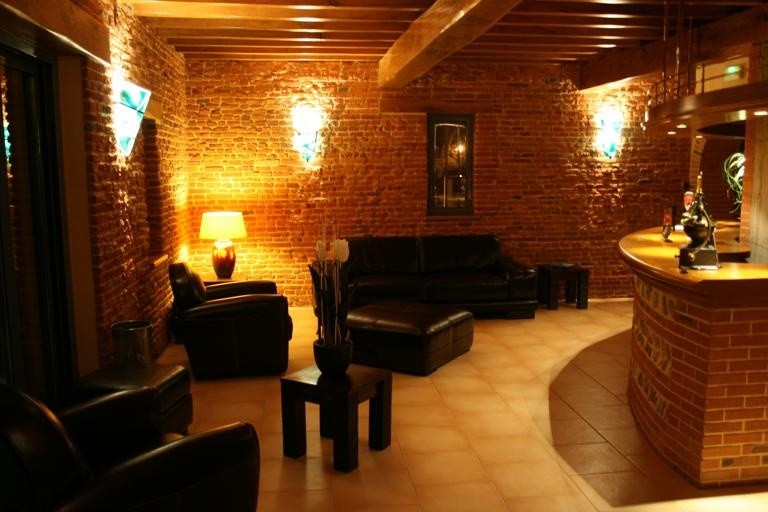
[346,301,472,373]
[73,365,194,441]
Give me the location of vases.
[310,340,352,376]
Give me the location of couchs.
[334,233,540,323]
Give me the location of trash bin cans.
[111,320,153,368]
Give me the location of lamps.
[112,78,152,157]
[199,210,249,279]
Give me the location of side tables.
[538,263,589,310]
[279,363,393,473]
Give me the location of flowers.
[307,208,351,345]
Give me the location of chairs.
[1,379,260,512]
[169,263,293,380]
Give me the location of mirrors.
[434,122,468,210]
